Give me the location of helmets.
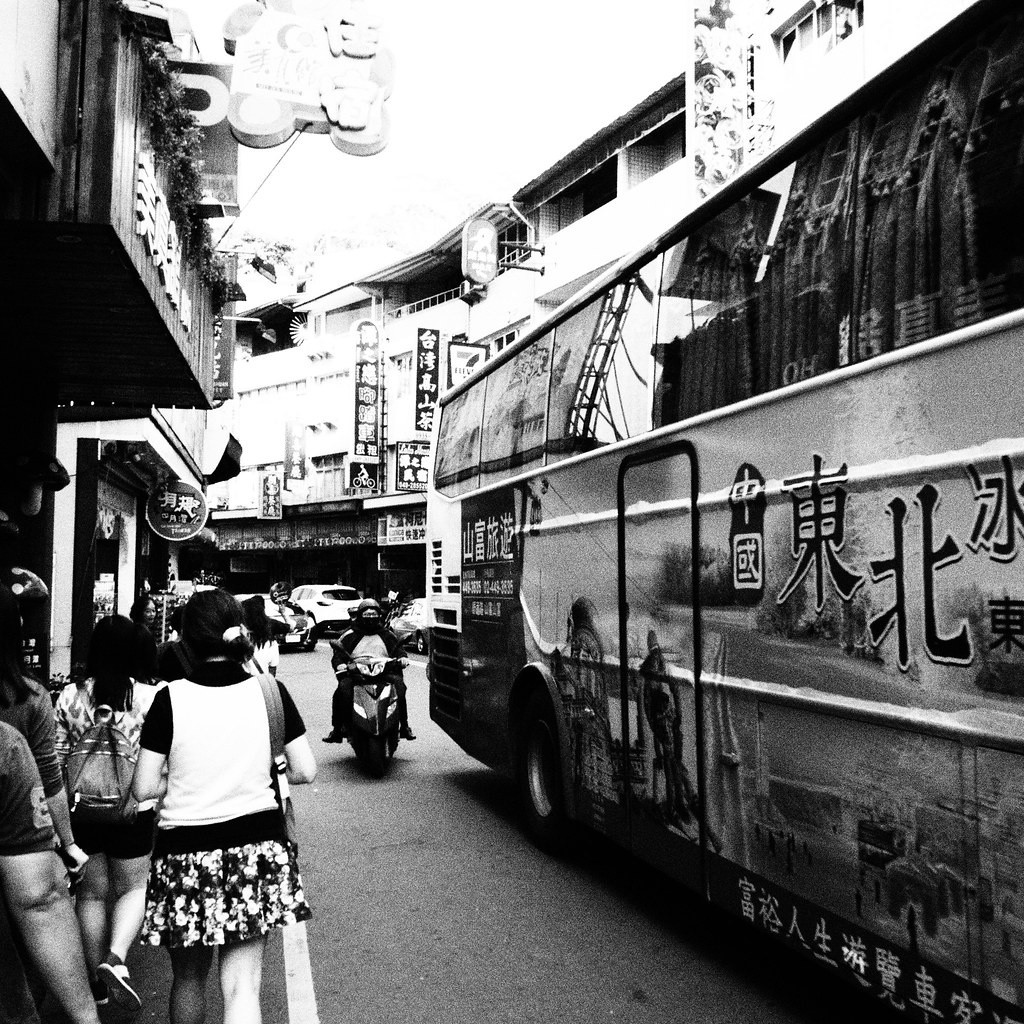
[359,598,381,612]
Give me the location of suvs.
[289,584,363,638]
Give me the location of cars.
[387,597,428,655]
[235,592,319,650]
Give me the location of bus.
[420,1,1024,1023]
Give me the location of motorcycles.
[329,631,414,778]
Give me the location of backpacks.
[65,682,139,819]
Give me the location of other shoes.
[400,727,416,740]
[322,731,342,743]
[90,988,109,1004]
[96,950,141,1009]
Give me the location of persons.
[0,566,319,1024]
[321,597,419,743]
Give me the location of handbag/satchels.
[256,674,296,842]
